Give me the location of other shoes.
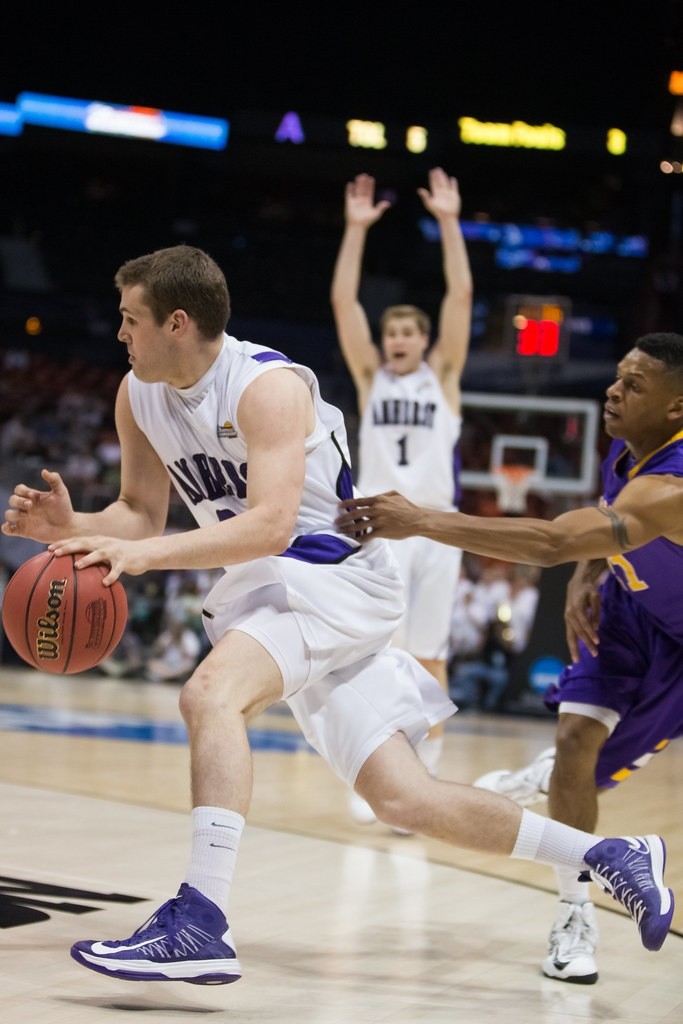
[351,790,378,826]
[386,823,414,837]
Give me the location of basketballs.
[0,548,130,678]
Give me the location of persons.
[105,568,213,682]
[0,240,674,992]
[458,550,542,713]
[330,326,679,982]
[323,163,479,835]
[0,356,122,512]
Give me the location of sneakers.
[541,901,599,985]
[473,747,556,808]
[578,834,674,951]
[71,883,243,985]
